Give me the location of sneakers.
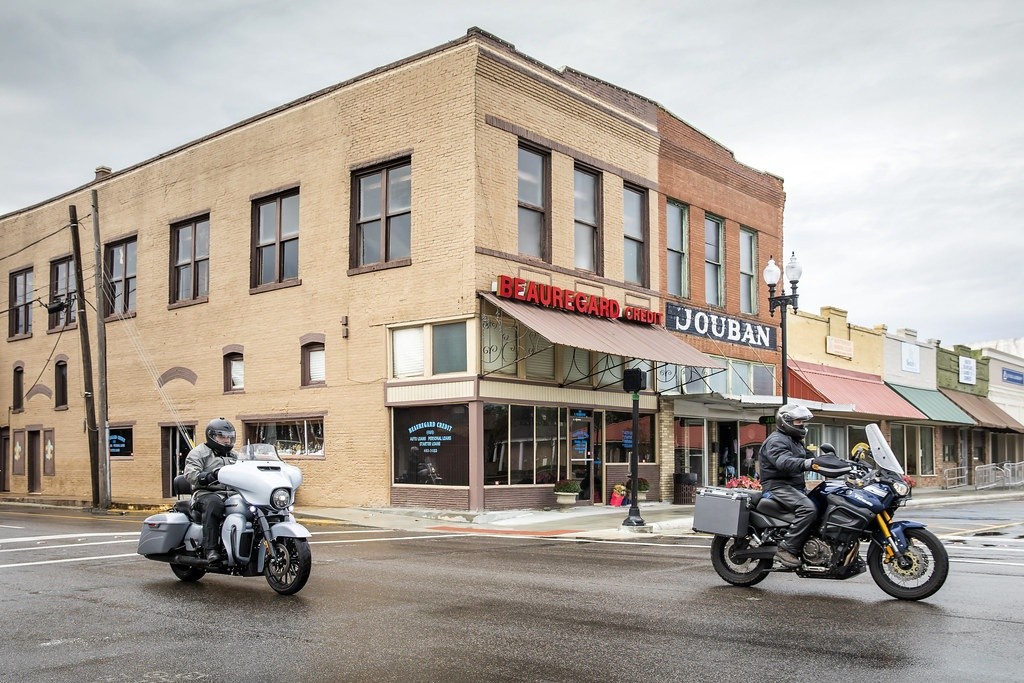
[772,547,802,567]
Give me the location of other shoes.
[206,549,220,564]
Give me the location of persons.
[184,418,238,566]
[758,402,816,568]
[405,446,426,483]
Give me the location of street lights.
[762,250,801,404]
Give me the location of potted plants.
[627,479,650,500]
[553,481,582,504]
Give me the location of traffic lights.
[622,368,648,392]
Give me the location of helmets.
[776,404,808,441]
[205,419,236,456]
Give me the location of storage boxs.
[691,486,750,538]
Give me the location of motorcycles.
[136,443,313,595]
[691,422,950,601]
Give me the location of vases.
[610,494,624,505]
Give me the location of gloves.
[198,471,220,486]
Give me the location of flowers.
[613,484,626,496]
[725,475,762,489]
[904,476,916,488]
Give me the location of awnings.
[477,290,728,369]
[787,360,1024,433]
